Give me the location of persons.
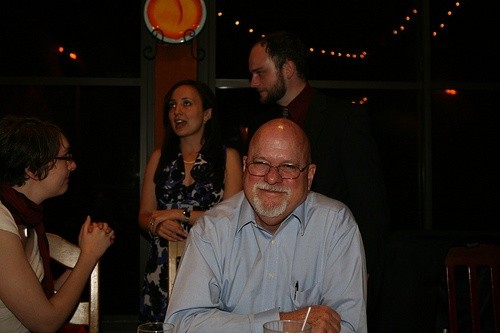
[139,79,244,324]
[239,31,390,248]
[0,116,116,333]
[163,118,368,333]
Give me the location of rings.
[150,222,154,226]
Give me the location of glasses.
[246,161,310,179]
[53,151,73,165]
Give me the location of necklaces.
[184,160,195,164]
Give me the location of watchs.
[183,210,191,223]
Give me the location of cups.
[137,322,175,333]
[262,319,313,333]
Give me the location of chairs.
[53,241,500,333]
